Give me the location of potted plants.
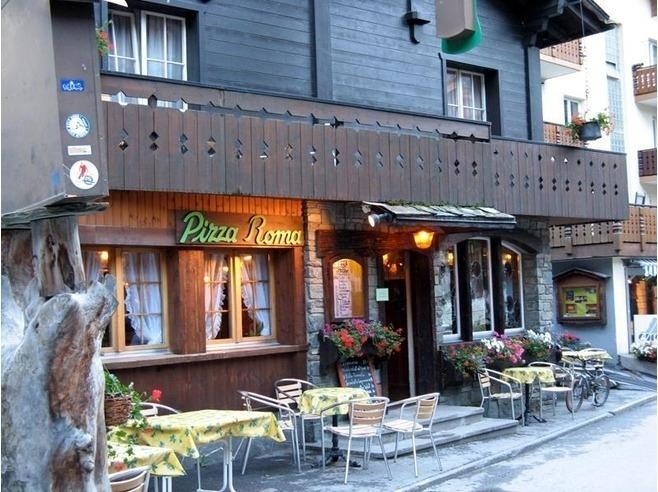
[104,372,139,426]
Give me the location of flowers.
[446,329,575,365]
[319,319,404,359]
[630,340,656,362]
[569,108,614,140]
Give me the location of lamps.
[367,213,387,227]
[413,231,434,248]
[97,250,110,273]
[447,250,453,269]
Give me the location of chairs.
[232,390,300,475]
[320,397,392,484]
[526,362,573,422]
[381,393,442,477]
[109,466,150,492]
[273,378,327,462]
[477,368,524,426]
[131,402,180,417]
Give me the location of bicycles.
[565,355,611,413]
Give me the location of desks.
[106,440,186,492]
[560,347,612,372]
[106,409,286,492]
[297,387,371,469]
[499,367,555,426]
